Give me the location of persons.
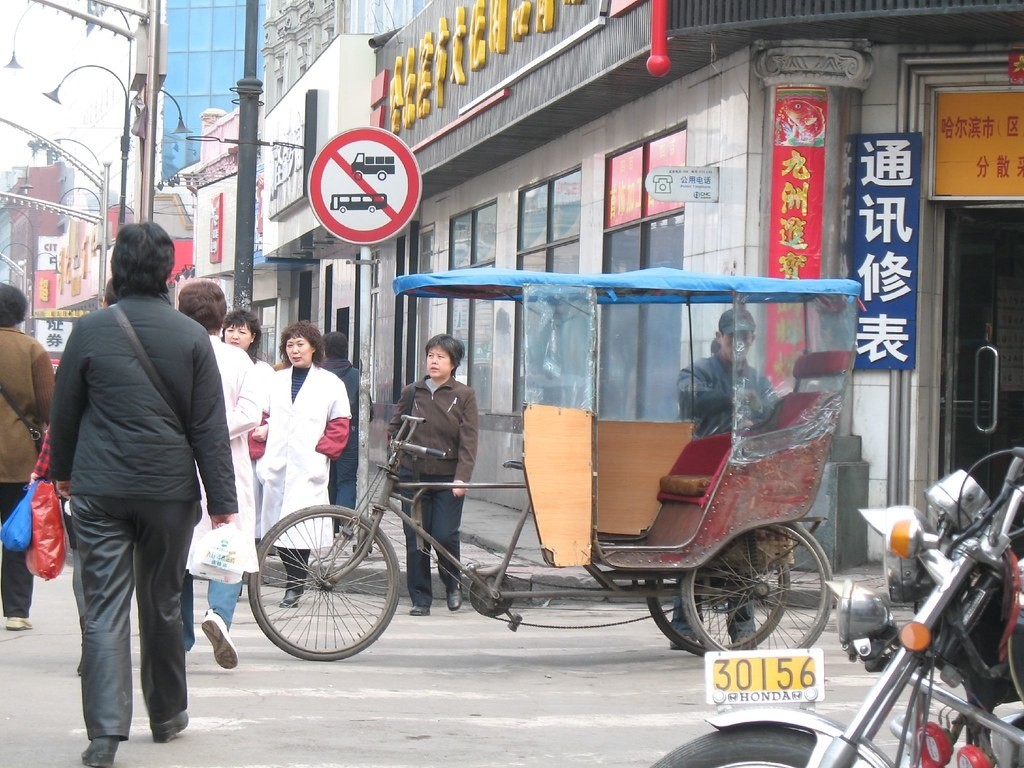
[667,307,780,652]
[221,310,276,539]
[177,277,263,670]
[253,318,353,608]
[388,334,480,616]
[27,276,119,679]
[317,331,373,540]
[55,219,240,768]
[0,282,56,632]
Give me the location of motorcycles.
[648,445,1024,768]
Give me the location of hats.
[719,308,756,335]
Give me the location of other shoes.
[671,635,697,650]
[731,630,755,643]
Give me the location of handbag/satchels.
[0,479,39,552]
[25,480,67,581]
[190,521,260,584]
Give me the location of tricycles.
[248,268,862,662]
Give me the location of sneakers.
[202,609,238,669]
[6,617,34,630]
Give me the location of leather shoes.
[281,589,304,608]
[83,737,119,767]
[447,583,462,612]
[150,711,189,743]
[409,606,430,616]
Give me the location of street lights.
[0,0,193,336]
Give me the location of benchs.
[656,350,852,508]
